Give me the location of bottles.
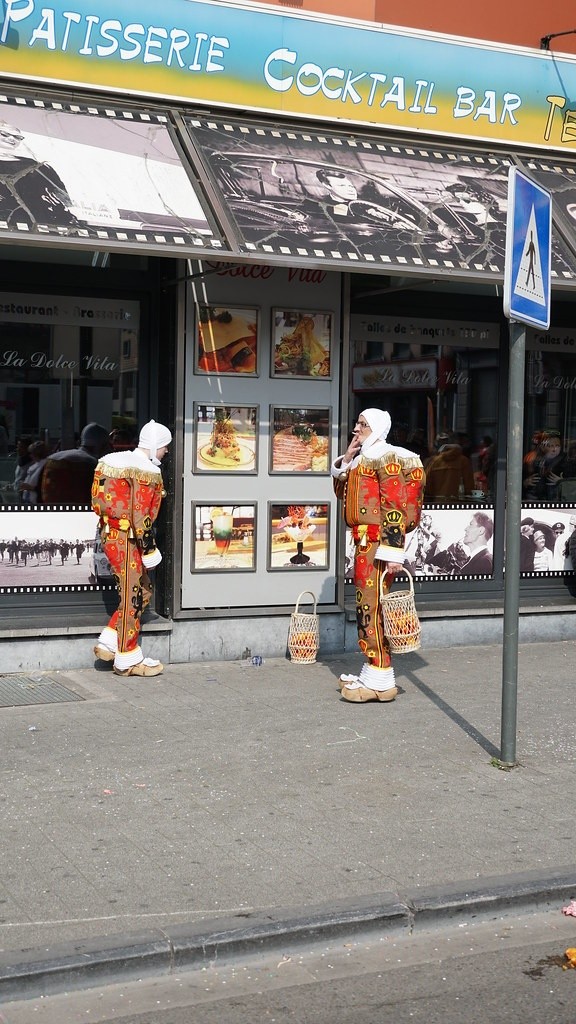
[459,477,465,501]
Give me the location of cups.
[471,490,484,497]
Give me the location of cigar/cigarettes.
[352,431,356,434]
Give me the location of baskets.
[288,591,320,664]
[380,567,421,655]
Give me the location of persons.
[331,407,427,703]
[422,428,566,502]
[0,120,79,226]
[434,182,507,255]
[91,419,172,678]
[316,169,423,232]
[425,511,494,575]
[520,514,576,570]
[0,536,90,567]
[4,422,111,505]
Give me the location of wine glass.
[213,515,233,558]
[283,525,316,564]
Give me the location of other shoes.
[114,646,163,676]
[339,662,399,702]
[94,626,120,661]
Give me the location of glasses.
[355,421,370,429]
[556,532,562,534]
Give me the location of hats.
[553,522,564,530]
[81,423,109,449]
[138,418,172,451]
[533,530,545,542]
[26,440,44,456]
[520,516,534,526]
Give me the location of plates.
[199,441,255,468]
[469,496,485,499]
